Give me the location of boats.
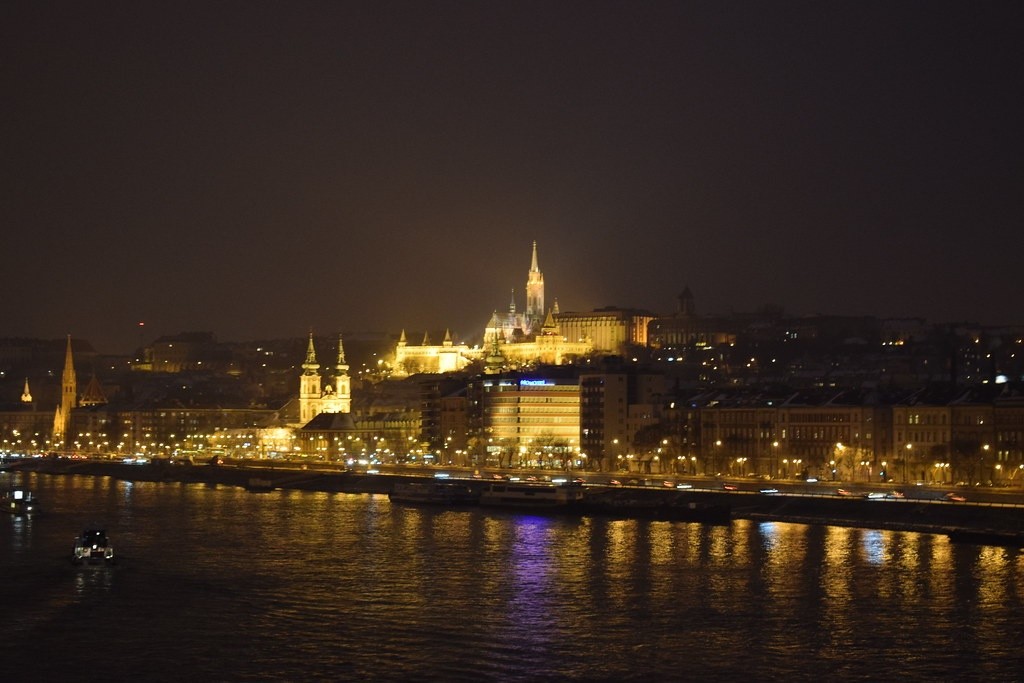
[0,483,44,517]
[72,527,113,562]
[480,478,586,513]
[389,483,474,507]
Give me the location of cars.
[838,488,965,503]
[433,472,779,494]
[365,468,379,475]
[0,452,223,467]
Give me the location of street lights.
[903,442,913,483]
[769,441,780,478]
[659,439,669,472]
[609,439,618,471]
[833,441,842,480]
[713,438,722,475]
[979,443,989,485]
